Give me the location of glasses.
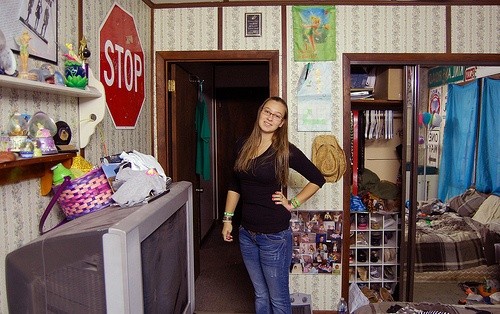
[262,107,283,121]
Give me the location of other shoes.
[384,216,396,229]
[357,267,368,281]
[385,248,396,263]
[357,232,368,246]
[384,266,395,280]
[358,250,367,262]
[370,266,381,279]
[349,232,356,246]
[371,218,380,229]
[372,249,380,262]
[349,266,355,280]
[371,232,381,246]
[350,217,356,230]
[349,249,355,264]
[358,214,369,229]
[385,232,395,246]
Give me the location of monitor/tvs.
[4,180,198,314]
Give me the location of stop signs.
[99,2,145,130]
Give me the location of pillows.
[472,195,500,235]
[448,187,488,216]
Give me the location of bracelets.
[291,196,301,208]
[222,212,234,222]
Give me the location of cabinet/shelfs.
[0,74,102,168]
[348,211,400,298]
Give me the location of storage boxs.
[375,66,404,100]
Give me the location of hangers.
[365,110,393,139]
[198,82,206,103]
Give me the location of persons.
[14,31,37,71]
[25,0,34,22]
[222,97,326,314]
[289,212,341,274]
[40,6,49,37]
[67,43,79,61]
[34,0,42,29]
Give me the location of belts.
[351,110,365,196]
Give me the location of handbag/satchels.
[38,166,113,234]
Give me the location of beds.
[405,186,500,272]
